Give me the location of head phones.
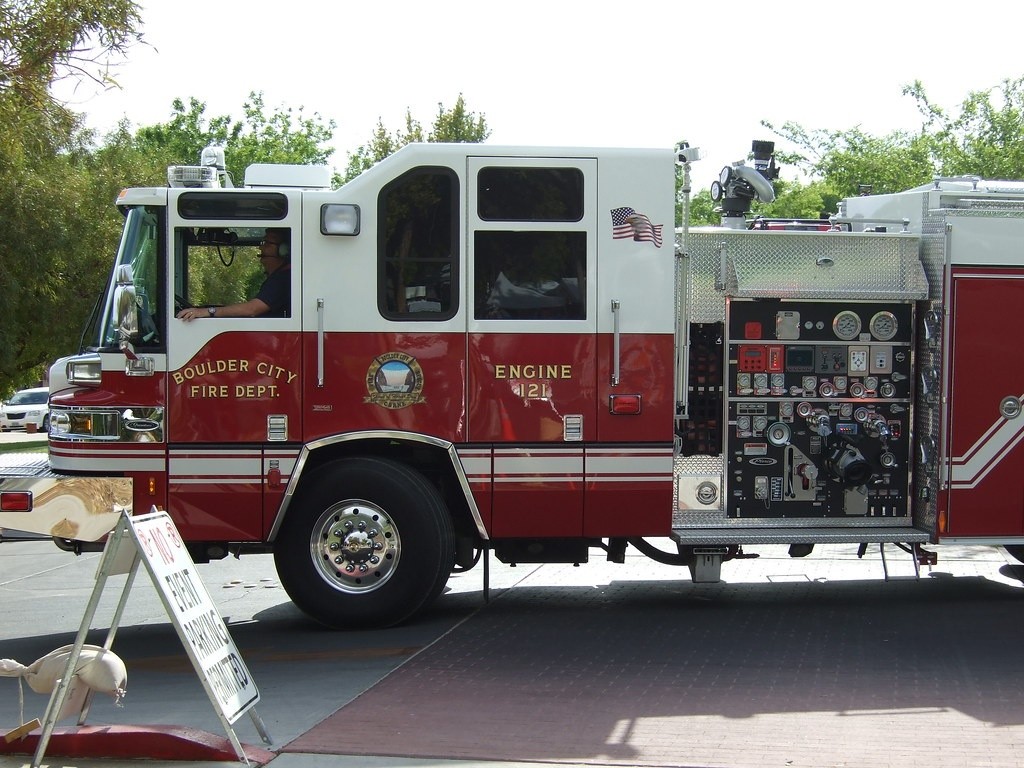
[278,238,291,258]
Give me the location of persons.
[176,230,291,322]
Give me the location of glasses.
[259,240,278,246]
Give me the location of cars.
[1,387,51,434]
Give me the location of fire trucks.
[41,135,1023,632]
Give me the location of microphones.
[257,254,288,259]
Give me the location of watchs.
[208,307,216,317]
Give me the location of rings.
[190,311,193,314]
